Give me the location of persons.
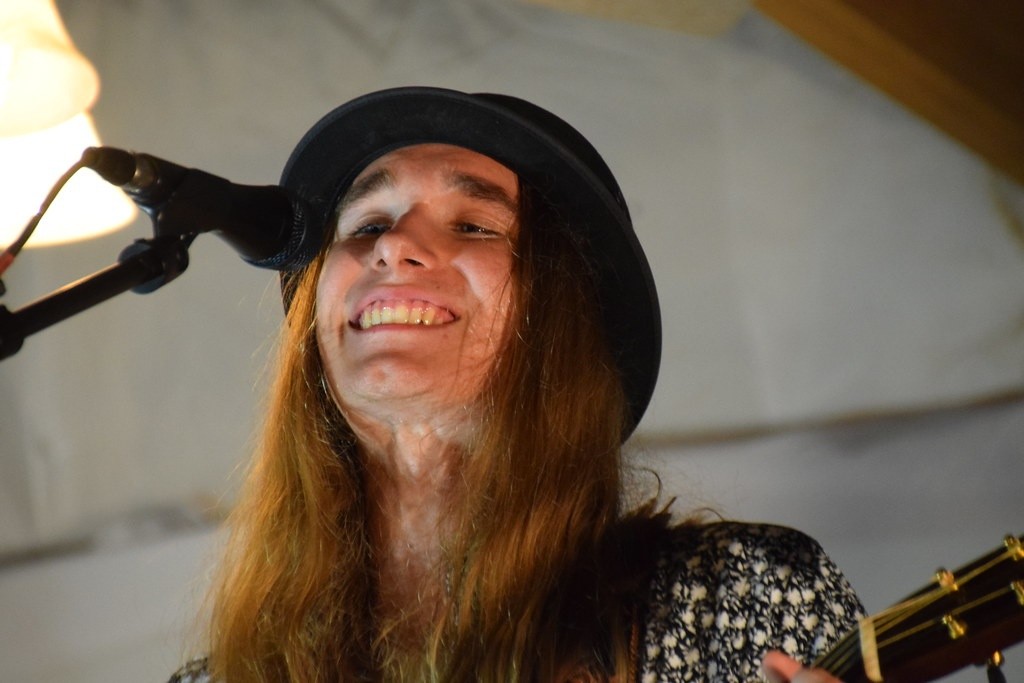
[169,85,891,683]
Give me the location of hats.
[278,84,664,447]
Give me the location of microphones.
[82,148,323,269]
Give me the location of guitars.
[817,532,1024,682]
